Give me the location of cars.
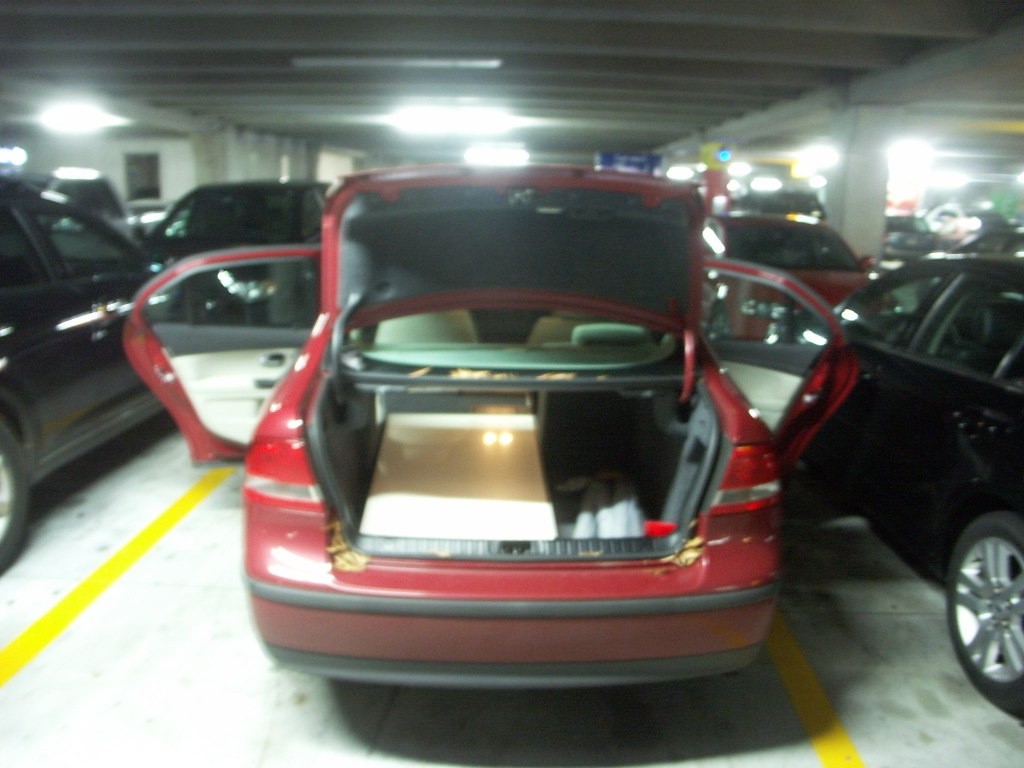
[702,178,1024,343]
[0,173,183,573]
[121,196,176,239]
[22,169,134,243]
[771,247,1024,718]
[129,177,327,320]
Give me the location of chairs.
[371,308,477,345]
[526,308,627,343]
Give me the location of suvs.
[122,167,862,695]
